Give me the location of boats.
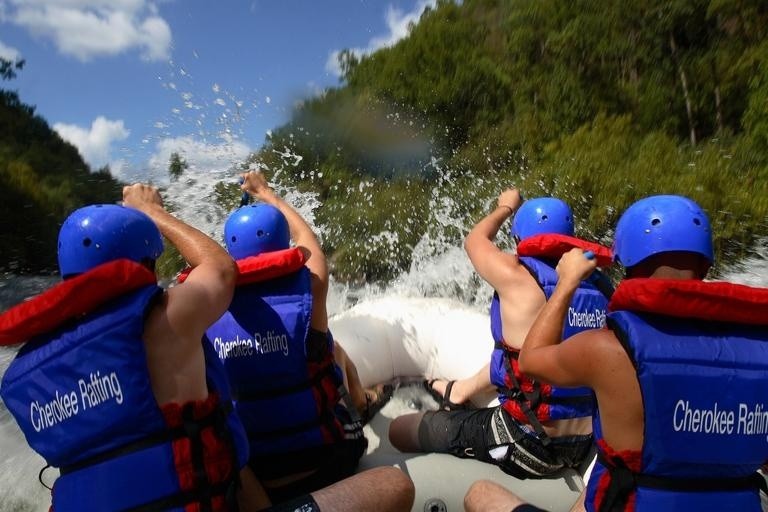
[328,298,586,512]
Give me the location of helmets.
[224,205,290,262]
[612,195,714,268]
[511,197,575,243]
[56,205,164,277]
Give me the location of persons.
[462,195,768,510]
[0,184,416,512]
[171,169,397,505]
[388,187,618,481]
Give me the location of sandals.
[424,377,469,411]
[355,382,394,427]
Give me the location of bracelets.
[495,204,514,215]
